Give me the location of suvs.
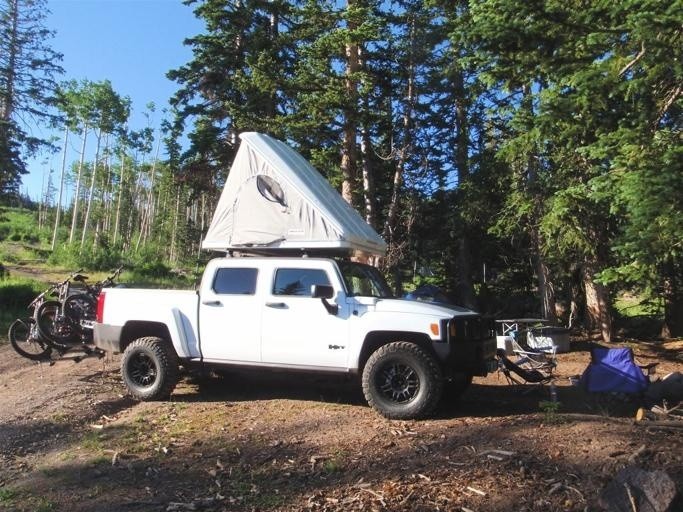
[92,255,499,421]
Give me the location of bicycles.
[63,264,126,329]
[35,269,117,348]
[10,286,59,359]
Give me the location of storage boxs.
[527,326,569,353]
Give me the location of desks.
[495,318,549,346]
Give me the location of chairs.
[497,347,556,393]
[509,331,557,374]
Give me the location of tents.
[578,347,647,393]
[405,284,450,304]
[202,132,387,255]
